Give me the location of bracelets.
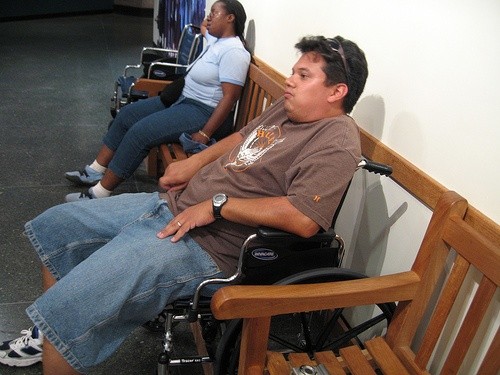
[198,130,210,139]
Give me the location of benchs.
[137,58,286,177]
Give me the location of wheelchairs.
[110,24,203,116]
[140,158,396,375]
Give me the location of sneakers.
[0,324,45,368]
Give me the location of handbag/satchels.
[160,76,185,108]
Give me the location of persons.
[0,35,368,375]
[63,0,251,203]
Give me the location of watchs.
[212,193,228,221]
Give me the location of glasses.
[325,37,353,91]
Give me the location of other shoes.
[65,164,105,186]
[65,186,97,202]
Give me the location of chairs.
[212,190,500,375]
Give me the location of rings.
[178,221,182,227]
